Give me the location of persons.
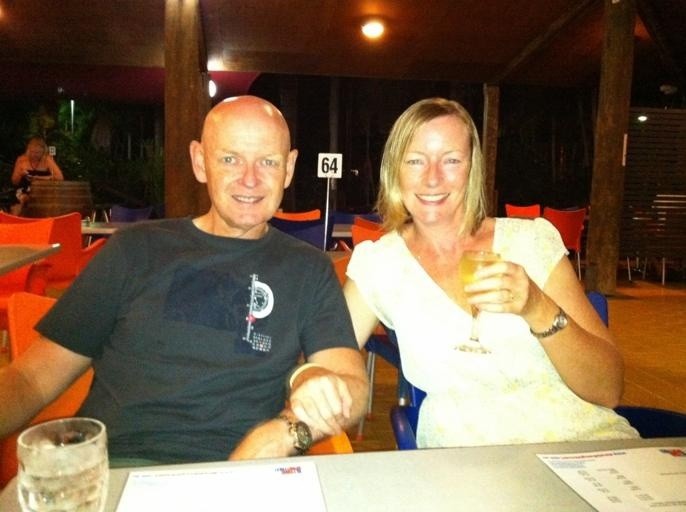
[289,97,643,449]
[11,139,64,216]
[0,95,369,468]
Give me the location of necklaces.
[30,159,40,171]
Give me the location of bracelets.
[285,362,320,388]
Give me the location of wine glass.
[454,250,504,355]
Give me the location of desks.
[0,436,686,512]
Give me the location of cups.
[16,416,110,512]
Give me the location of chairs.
[1,182,685,486]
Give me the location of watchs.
[270,415,312,455]
[530,307,568,338]
